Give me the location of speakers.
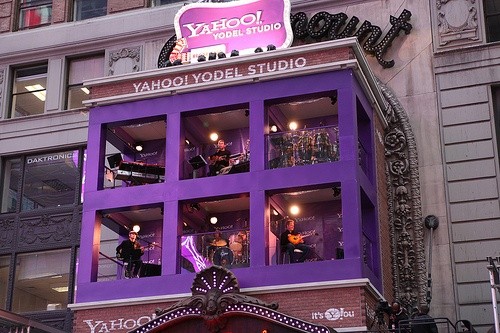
[137,263,161,278]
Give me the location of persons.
[209,230,229,247]
[280,220,311,264]
[116,231,145,278]
[207,140,230,177]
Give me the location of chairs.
[219,156,234,175]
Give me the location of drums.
[228,235,243,251]
[211,247,236,267]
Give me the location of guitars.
[287,228,319,246]
[206,140,233,167]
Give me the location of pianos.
[116,160,167,184]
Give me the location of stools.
[122,261,141,279]
